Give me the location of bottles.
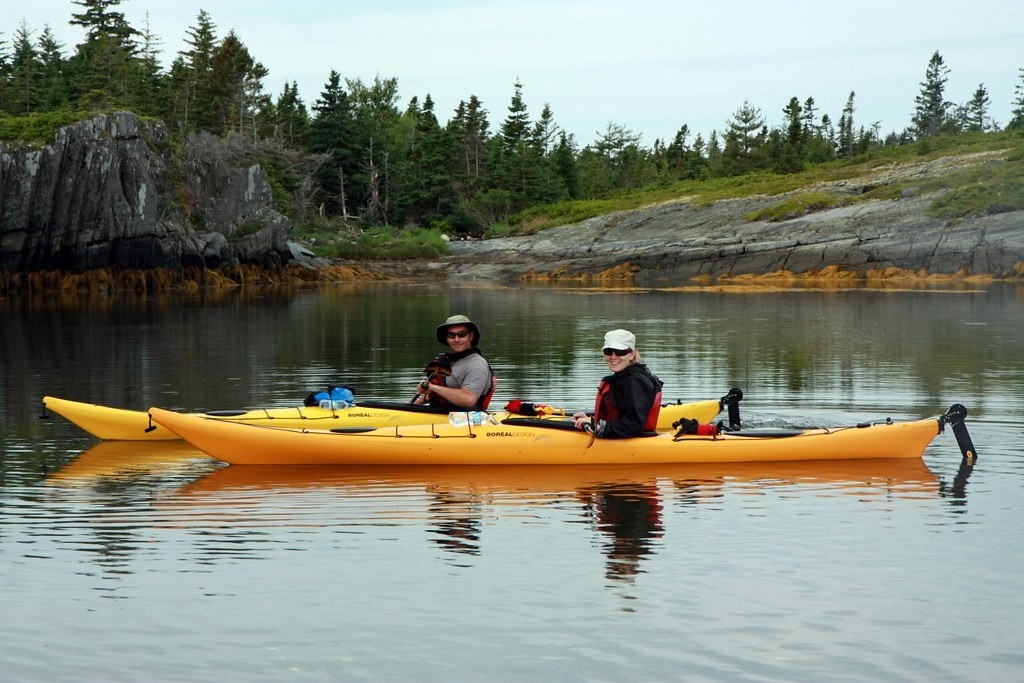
[319,400,356,410]
[448,412,492,426]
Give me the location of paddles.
[410,360,452,406]
[584,423,598,450]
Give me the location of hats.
[601,329,636,351]
[435,315,481,346]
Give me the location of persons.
[572,328,663,438]
[414,306,491,415]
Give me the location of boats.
[145,403,979,465]
[39,387,744,441]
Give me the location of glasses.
[604,348,632,356]
[446,329,471,339]
